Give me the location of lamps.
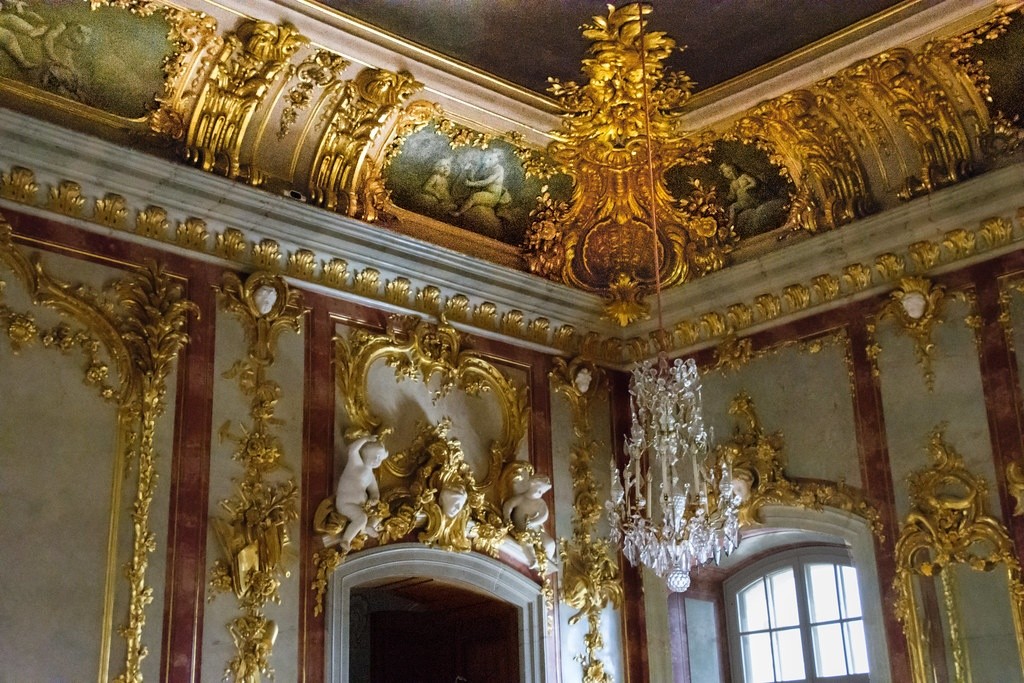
[605,0,742,593]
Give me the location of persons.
[439,485,467,518]
[418,152,457,213]
[448,148,506,218]
[335,436,388,550]
[717,161,758,227]
[1,1,90,82]
[502,472,555,571]
